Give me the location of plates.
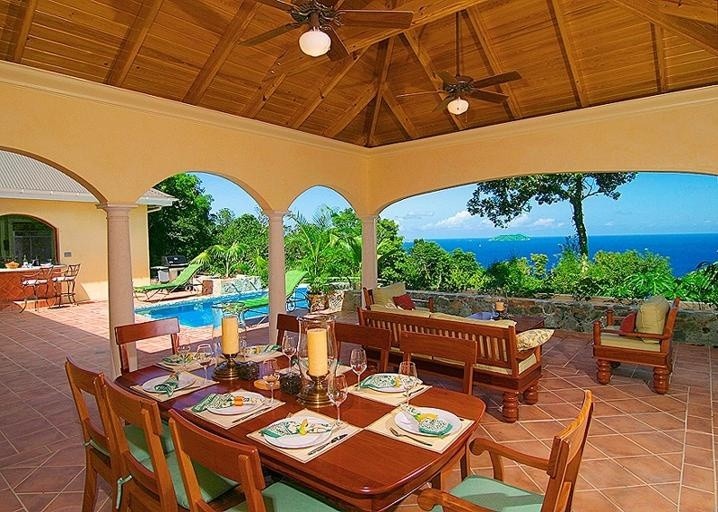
[206,390,265,415]
[367,373,419,393]
[264,416,331,448]
[143,374,196,394]
[394,408,460,436]
[241,346,278,359]
[293,359,302,372]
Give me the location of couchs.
[353,304,543,424]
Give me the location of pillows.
[516,327,556,351]
[392,293,416,310]
[618,310,637,336]
[371,281,406,305]
[635,293,669,343]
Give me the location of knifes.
[308,434,347,456]
[234,407,271,425]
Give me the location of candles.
[495,301,505,311]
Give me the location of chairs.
[132,259,207,303]
[50,263,81,307]
[362,284,434,311]
[593,296,681,395]
[216,269,309,327]
[18,265,55,313]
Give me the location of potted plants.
[281,206,357,312]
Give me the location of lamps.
[446,98,469,116]
[298,28,333,58]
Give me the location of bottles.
[23,254,29,267]
[297,311,340,409]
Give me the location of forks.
[388,428,433,448]
[402,386,425,398]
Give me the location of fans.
[237,0,414,63]
[395,11,522,114]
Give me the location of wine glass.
[263,358,281,407]
[237,327,247,360]
[176,334,191,372]
[350,348,367,394]
[400,361,417,407]
[196,345,212,384]
[211,325,223,352]
[282,336,298,377]
[326,374,349,428]
